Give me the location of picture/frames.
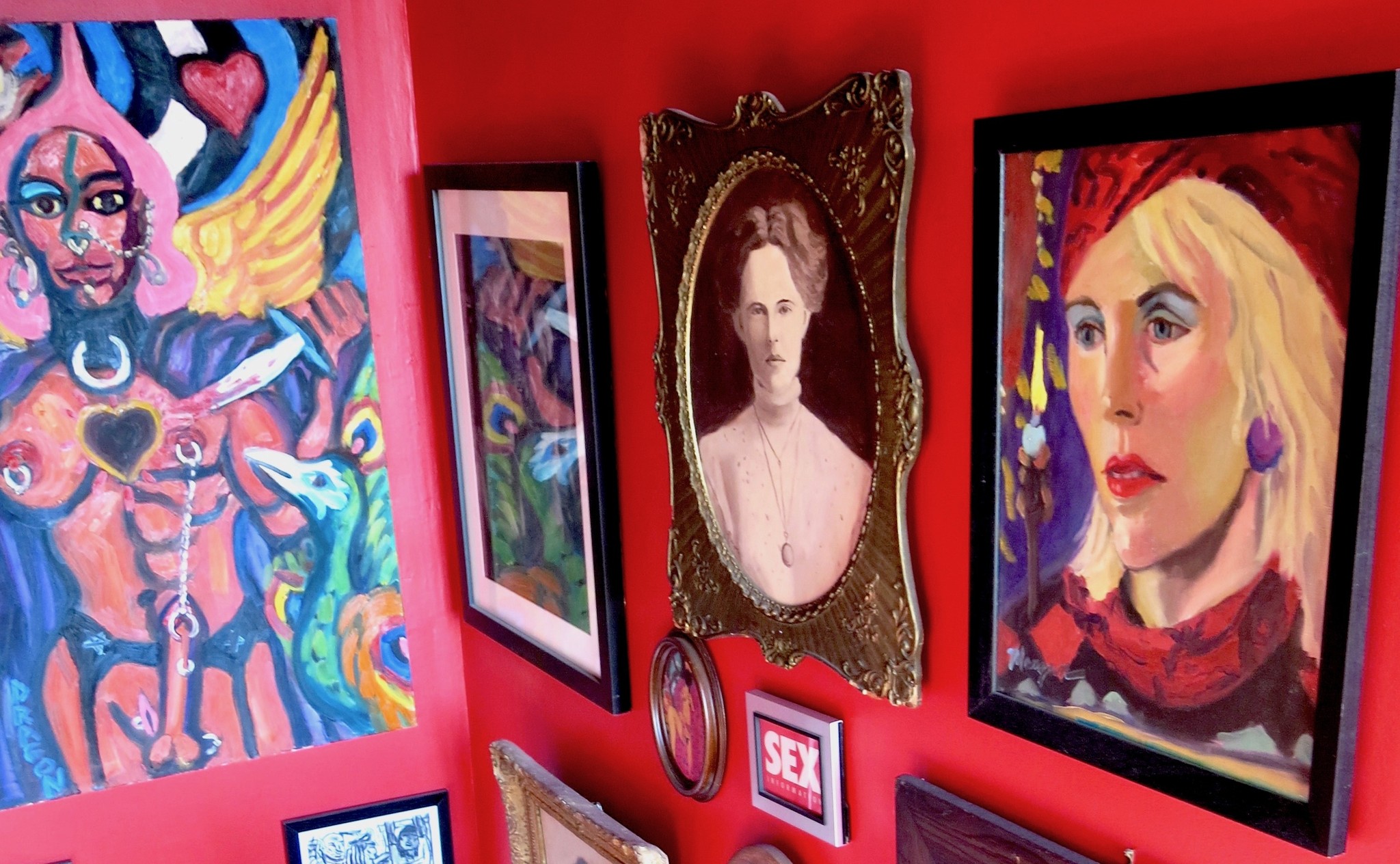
[488,739,668,864]
[421,160,629,717]
[641,68,926,710]
[283,788,456,864]
[648,630,726,802]
[966,62,1400,858]
